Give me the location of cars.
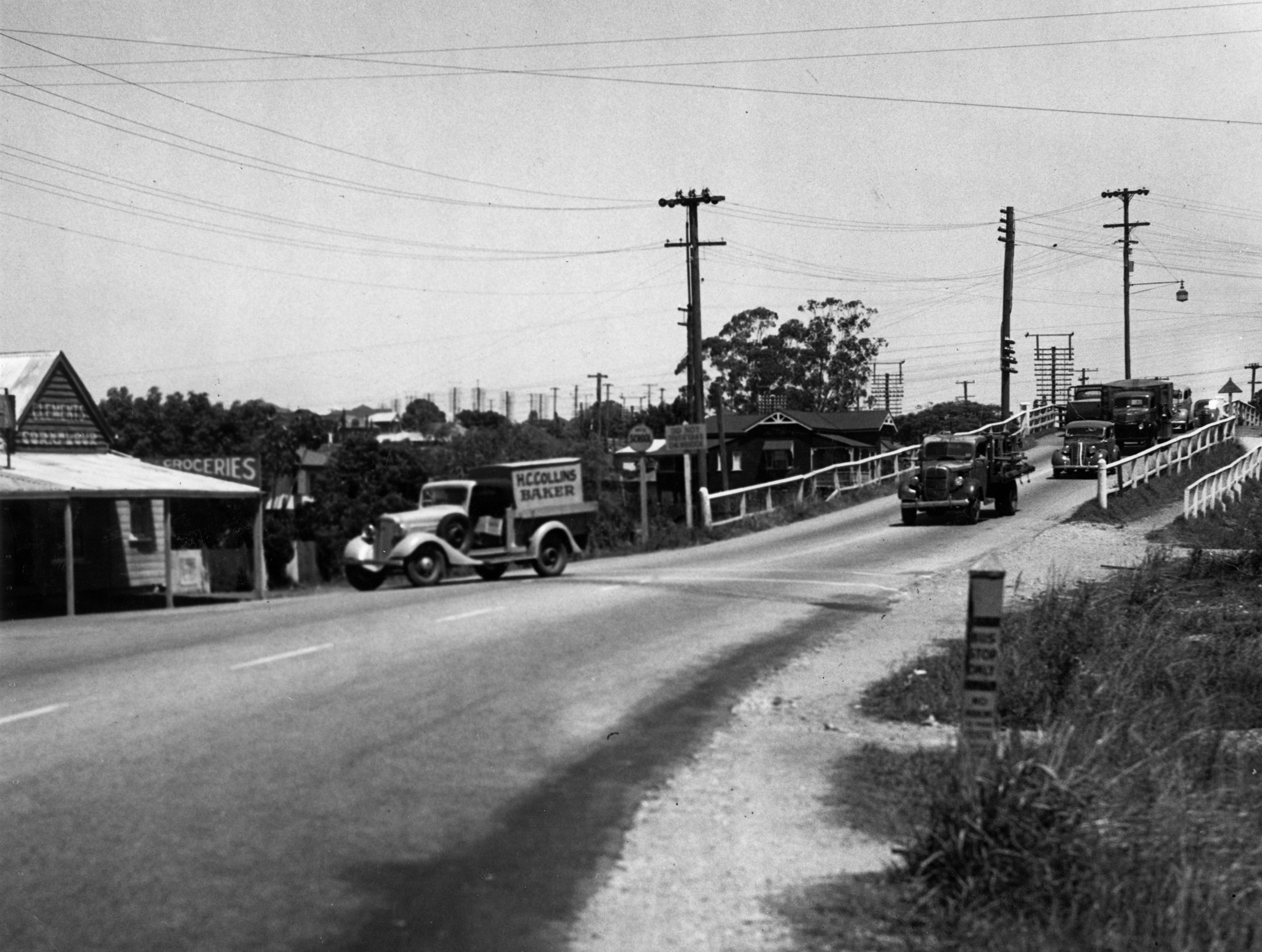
[1190,397,1230,428]
[1051,420,1122,479]
[1167,384,1196,433]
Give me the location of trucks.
[1065,375,1175,456]
[339,452,601,592]
[898,428,1036,525]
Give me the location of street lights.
[1124,278,1189,381]
